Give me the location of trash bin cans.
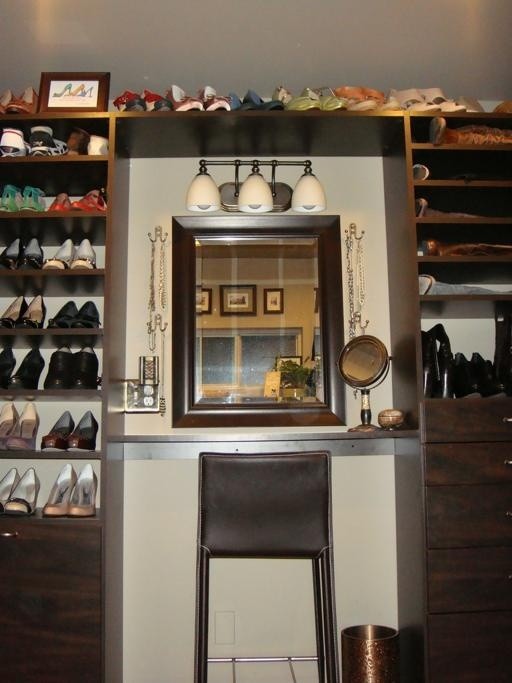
[341,624,400,683]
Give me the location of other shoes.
[70,190,107,212]
[0,126,110,156]
[7,85,39,113]
[43,239,75,270]
[1,238,25,270]
[47,193,72,212]
[1,90,15,112]
[0,184,23,212]
[492,102,510,113]
[22,238,44,269]
[70,239,97,270]
[19,186,46,212]
[113,85,485,113]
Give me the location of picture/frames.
[275,355,302,388]
[219,285,257,316]
[37,71,111,112]
[263,288,284,314]
[201,288,213,315]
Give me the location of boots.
[410,160,510,181]
[414,194,511,222]
[421,322,457,400]
[418,271,510,300]
[424,116,512,149]
[423,234,512,259]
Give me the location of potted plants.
[277,360,312,399]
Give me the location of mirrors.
[171,215,348,428]
[336,334,394,433]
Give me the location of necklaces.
[346,236,364,313]
[148,242,167,313]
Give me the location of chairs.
[194,449,339,683]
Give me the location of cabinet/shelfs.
[0,110,512,683]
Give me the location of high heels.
[1,466,21,515]
[66,463,98,517]
[52,85,72,97]
[2,402,20,451]
[4,468,41,516]
[47,301,77,329]
[78,83,94,97]
[10,348,45,390]
[71,300,101,329]
[67,411,99,452]
[17,295,46,329]
[5,402,40,451]
[41,411,75,452]
[65,84,85,97]
[0,295,27,329]
[43,463,78,516]
[0,347,17,390]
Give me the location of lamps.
[186,159,326,215]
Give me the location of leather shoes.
[44,344,74,390]
[72,346,99,390]
[452,349,507,402]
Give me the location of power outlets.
[124,379,159,414]
